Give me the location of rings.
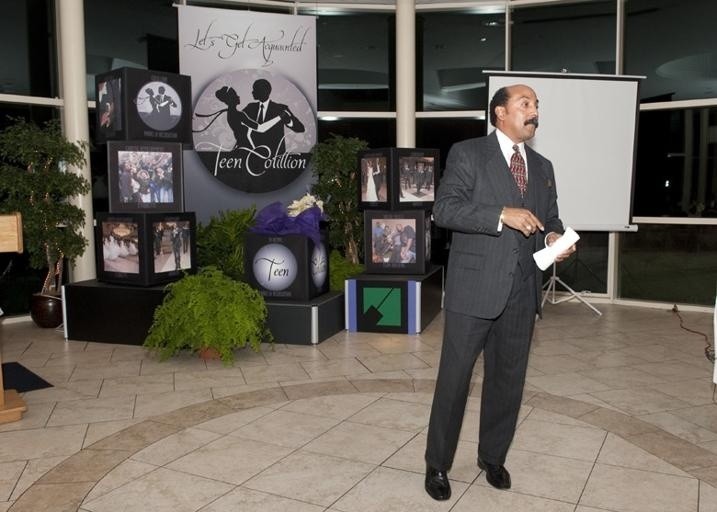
[526,224,532,232]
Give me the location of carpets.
[2,358,56,395]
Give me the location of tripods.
[535,259,605,321]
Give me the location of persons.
[214,84,290,154]
[118,152,173,204]
[240,78,304,153]
[421,82,574,501]
[152,85,178,120]
[100,216,191,273]
[145,89,173,120]
[361,156,435,265]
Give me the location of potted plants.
[138,265,277,370]
[0,109,95,332]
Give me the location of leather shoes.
[477,455,512,489]
[424,465,452,501]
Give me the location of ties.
[509,145,528,196]
